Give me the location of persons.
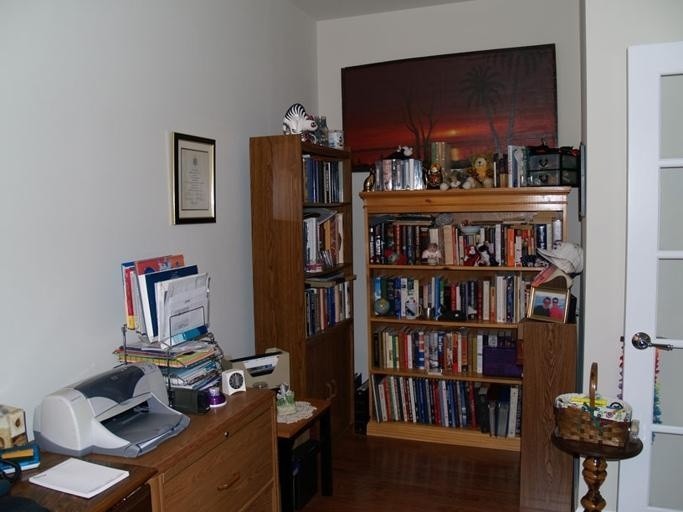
[534,297,551,317]
[550,297,562,322]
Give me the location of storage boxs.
[526,153,580,187]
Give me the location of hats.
[537,241,585,276]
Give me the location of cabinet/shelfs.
[250,134,355,447]
[0,382,282,512]
[519,321,576,511]
[358,186,571,452]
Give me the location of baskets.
[555,363,633,448]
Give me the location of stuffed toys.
[429,147,495,190]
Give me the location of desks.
[552,425,643,512]
[277,396,333,512]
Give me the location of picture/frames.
[526,285,570,324]
[174,131,216,225]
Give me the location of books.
[507,145,525,187]
[367,220,563,267]
[28,456,130,499]
[375,160,425,191]
[369,273,536,323]
[368,326,528,374]
[301,148,352,338]
[111,254,219,389]
[371,375,520,441]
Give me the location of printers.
[31,360,190,459]
